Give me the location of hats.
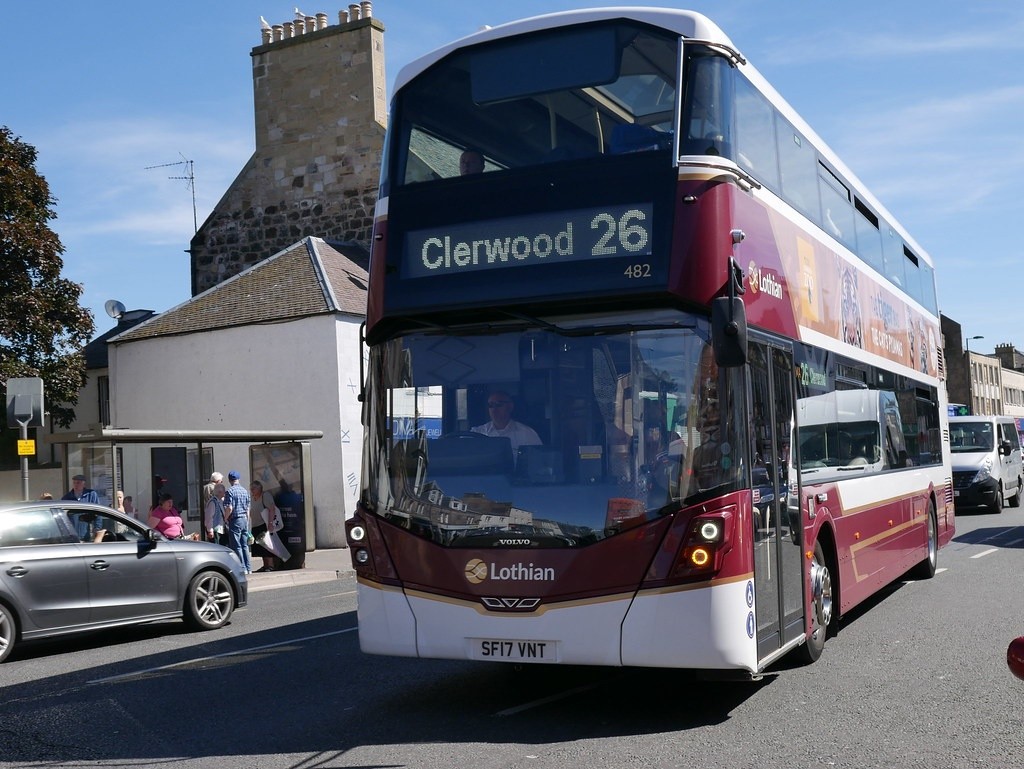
[72,475,86,481]
[228,470,240,481]
[40,493,53,500]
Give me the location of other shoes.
[257,566,266,572]
[265,566,275,572]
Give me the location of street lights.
[966,335,985,415]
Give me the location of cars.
[0,499,249,664]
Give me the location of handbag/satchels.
[260,491,284,533]
[246,531,254,546]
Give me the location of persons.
[41,493,53,500]
[61,476,102,539]
[95,529,108,542]
[460,148,485,175]
[147,493,182,540]
[706,131,724,142]
[116,491,129,532]
[826,207,841,237]
[80,515,86,521]
[460,392,542,467]
[203,471,301,574]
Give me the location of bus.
[343,6,958,682]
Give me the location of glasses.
[487,400,511,408]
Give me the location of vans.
[946,414,1024,514]
[789,389,912,480]
